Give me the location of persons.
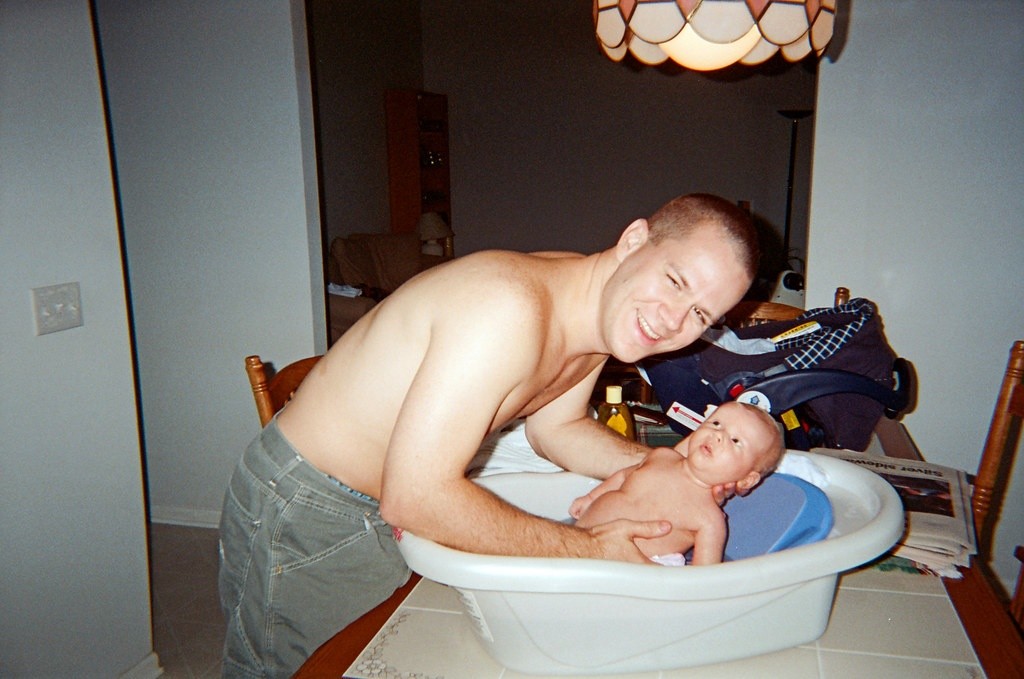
[216,187,763,679]
[564,401,786,567]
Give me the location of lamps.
[592,0,845,70]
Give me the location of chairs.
[960,336,1022,627]
[244,353,326,431]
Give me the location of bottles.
[421,151,442,167]
[599,385,637,443]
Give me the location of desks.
[289,420,989,679]
